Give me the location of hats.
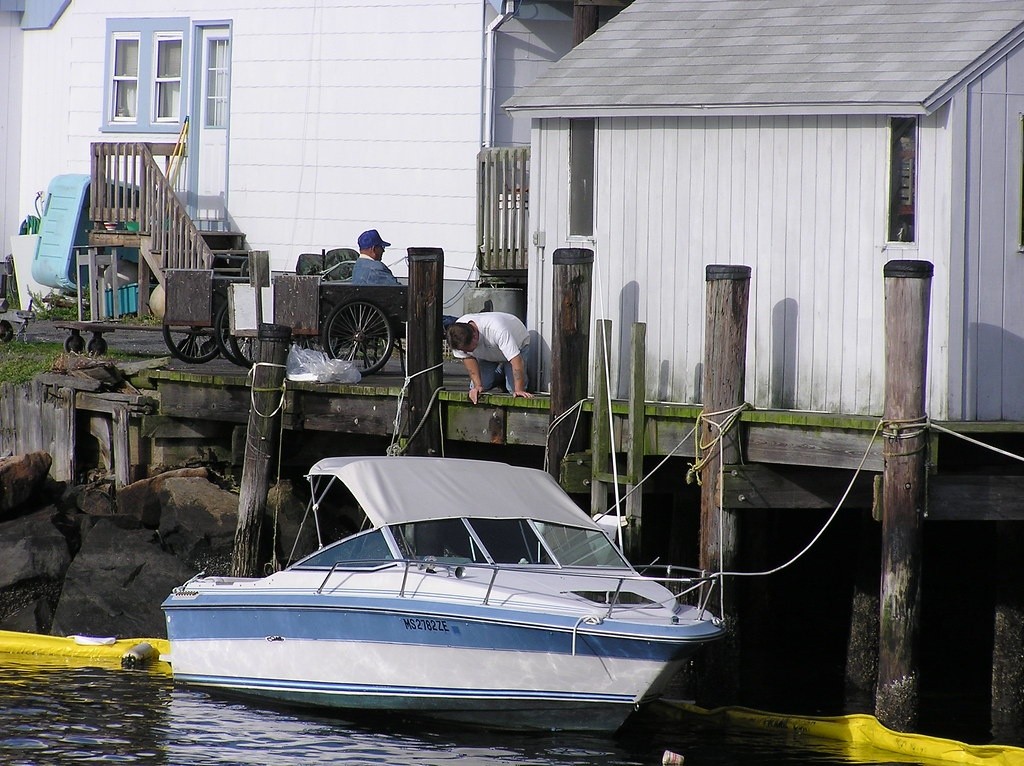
[358,230,391,249]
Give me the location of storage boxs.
[105,283,156,318]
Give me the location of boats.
[159,454,727,738]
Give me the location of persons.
[446,310,537,405]
[349,229,406,286]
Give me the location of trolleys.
[162,266,263,368]
[275,272,453,378]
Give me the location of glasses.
[375,244,387,251]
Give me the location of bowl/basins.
[125,222,139,231]
[104,222,117,231]
